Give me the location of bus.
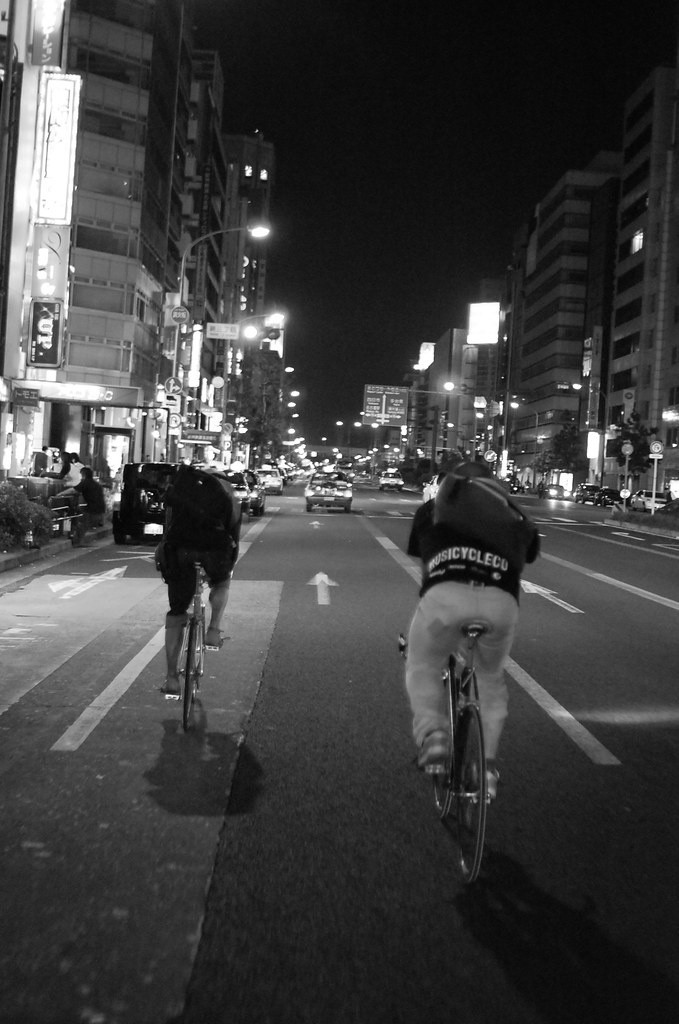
[334,458,354,483]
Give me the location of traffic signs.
[363,384,408,428]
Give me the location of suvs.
[112,462,182,544]
[574,484,600,505]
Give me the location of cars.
[305,471,355,513]
[631,489,669,512]
[379,470,405,490]
[545,484,571,500]
[258,465,301,494]
[594,488,625,509]
[507,478,523,493]
[221,469,265,516]
[421,474,443,502]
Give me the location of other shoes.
[159,677,183,695]
[471,763,502,801]
[415,731,449,769]
[205,630,231,650]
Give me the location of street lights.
[162,222,270,461]
[219,310,284,465]
[512,402,539,490]
[572,382,608,486]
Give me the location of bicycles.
[163,568,218,731]
[429,622,490,891]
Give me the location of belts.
[457,579,491,587]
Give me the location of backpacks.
[159,462,235,556]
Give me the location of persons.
[40,451,106,547]
[515,477,548,499]
[406,464,540,800]
[155,468,243,694]
